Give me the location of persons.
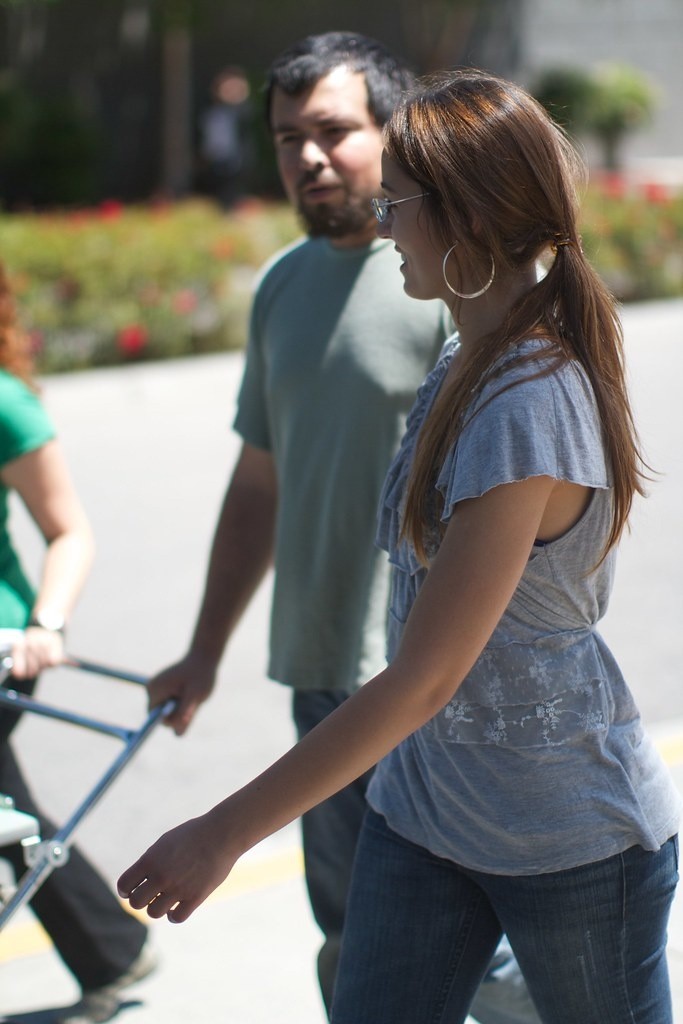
[0,257,160,1022]
[114,73,680,1023]
[156,35,545,1024]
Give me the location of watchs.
[23,610,69,635]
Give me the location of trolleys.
[0,652,179,931]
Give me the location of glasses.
[368,190,431,223]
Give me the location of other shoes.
[59,944,159,1023]
